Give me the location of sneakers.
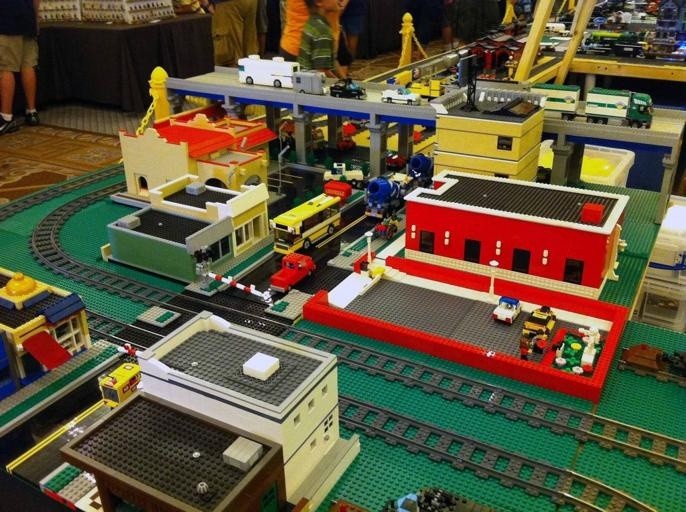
[0,114,18,135]
[24,112,40,126]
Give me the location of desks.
[38,11,213,118]
